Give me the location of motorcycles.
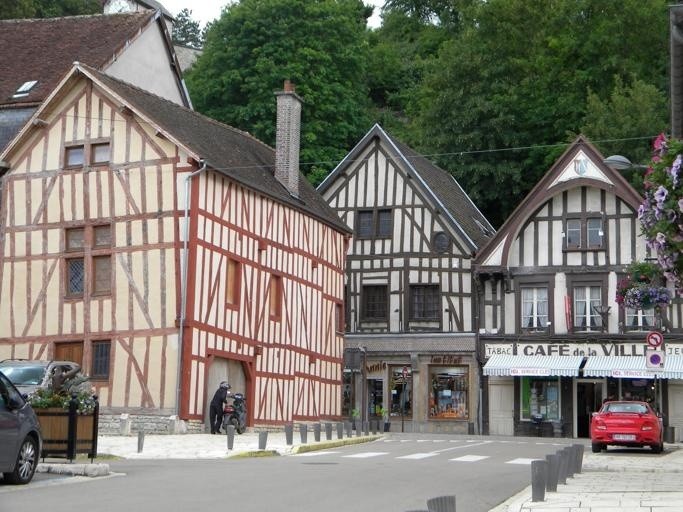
[221,392,248,434]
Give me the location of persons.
[209,380,230,430]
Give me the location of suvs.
[1,359,84,405]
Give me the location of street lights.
[601,153,651,173]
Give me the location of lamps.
[593,305,611,333]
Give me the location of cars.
[0,367,46,487]
[590,399,664,454]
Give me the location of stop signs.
[402,366,409,380]
